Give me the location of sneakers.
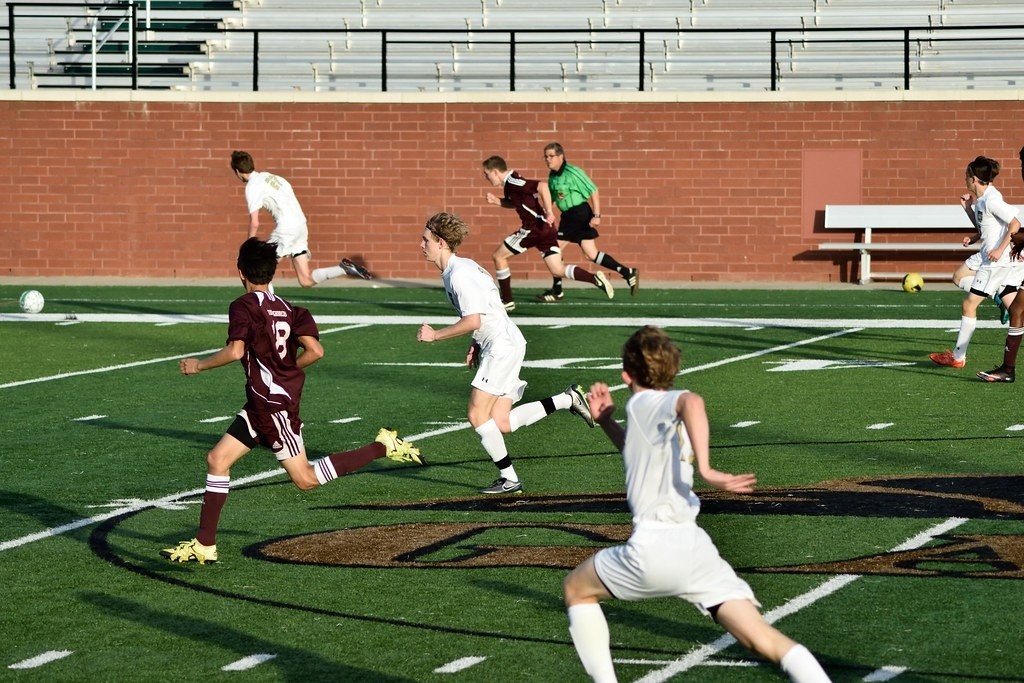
[159,538,218,564]
[623,267,639,297]
[376,426,428,467]
[536,290,564,302]
[976,367,1014,381]
[929,349,965,368]
[480,477,522,494]
[565,384,594,429]
[500,297,515,311]
[594,270,615,301]
[340,259,372,280]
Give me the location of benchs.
[0,0,1024,90]
[819,204,1024,283]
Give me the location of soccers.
[902,272,924,293]
[20,289,45,313]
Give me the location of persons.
[159,237,426,564]
[416,213,597,494]
[563,325,833,682]
[230,150,374,296]
[930,146,1024,382]
[482,155,615,312]
[536,142,639,301]
